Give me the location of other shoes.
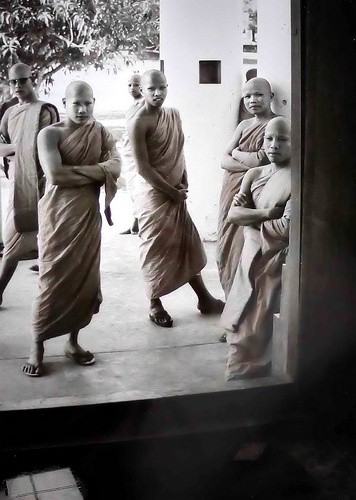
[219,332,226,342]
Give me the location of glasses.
[8,75,33,86]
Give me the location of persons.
[217,78,289,343]
[120,71,152,236]
[20,81,121,376]
[0,64,59,308]
[135,69,229,328]
[238,69,258,124]
[220,116,292,384]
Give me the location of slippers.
[22,360,43,377]
[63,347,95,365]
[201,299,227,313]
[148,310,173,327]
